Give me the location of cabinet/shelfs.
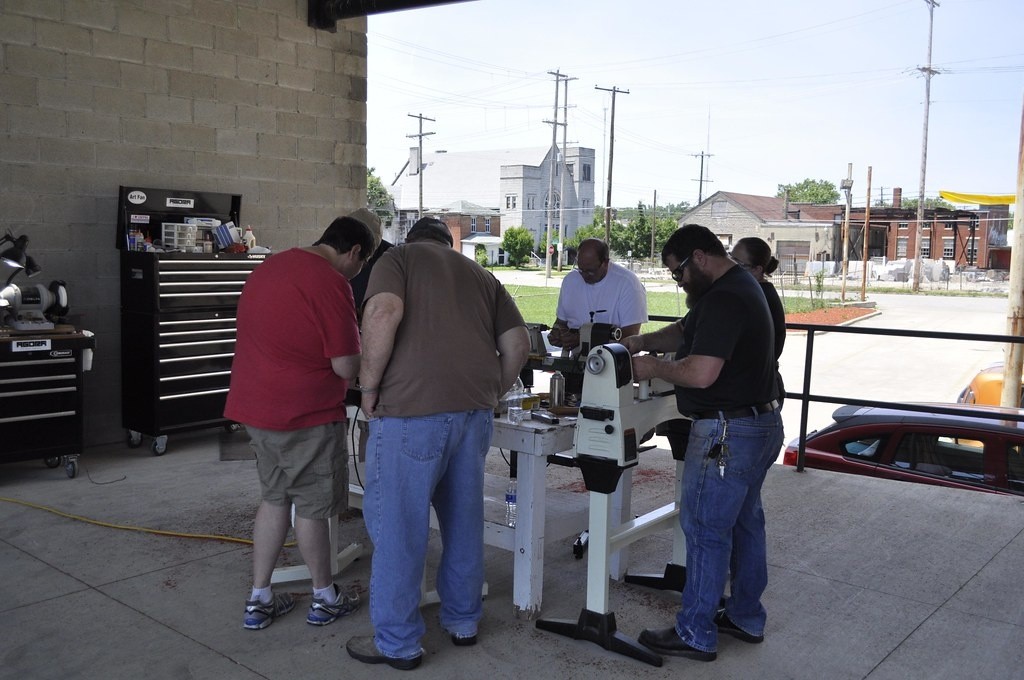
[116,185,273,455]
[159,222,196,249]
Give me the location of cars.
[783,401,1024,497]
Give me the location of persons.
[621,224,785,661]
[346,208,394,307]
[223,216,375,630]
[346,217,532,670]
[726,237,786,411]
[547,239,648,358]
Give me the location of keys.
[703,443,730,479]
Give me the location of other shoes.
[449,632,477,646]
[346,635,423,671]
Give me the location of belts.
[687,398,781,420]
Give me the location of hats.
[343,207,384,248]
[405,217,454,248]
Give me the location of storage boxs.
[212,220,241,249]
[494,395,540,413]
[185,217,215,226]
[0,334,96,478]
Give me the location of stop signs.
[548,245,554,255]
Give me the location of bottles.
[129,229,153,252]
[504,477,516,527]
[507,374,524,427]
[549,371,565,408]
[242,225,256,251]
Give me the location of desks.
[326,402,632,620]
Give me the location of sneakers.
[306,580,363,626]
[242,585,296,630]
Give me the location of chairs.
[1009,448,1024,491]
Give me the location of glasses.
[727,251,756,269]
[573,257,610,277]
[672,253,693,282]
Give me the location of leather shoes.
[714,607,764,644]
[636,625,717,662]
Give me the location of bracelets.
[359,385,378,393]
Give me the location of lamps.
[0,229,41,319]
